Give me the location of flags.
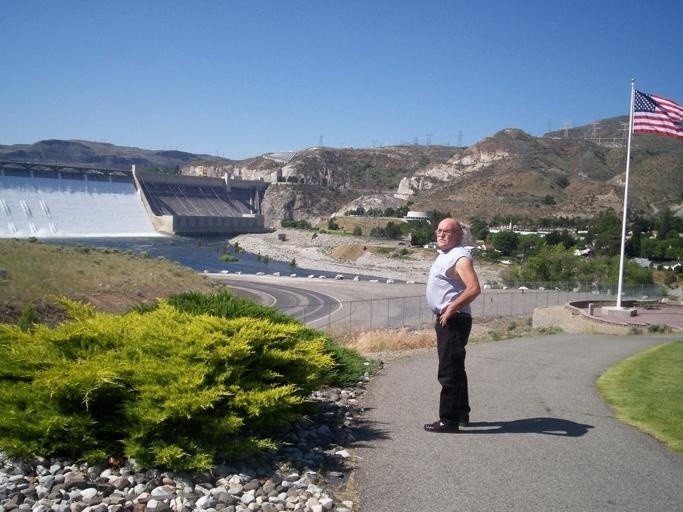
[634,90,683,143]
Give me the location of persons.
[422,217,480,432]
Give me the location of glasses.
[435,229,456,234]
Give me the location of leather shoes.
[424,419,469,433]
